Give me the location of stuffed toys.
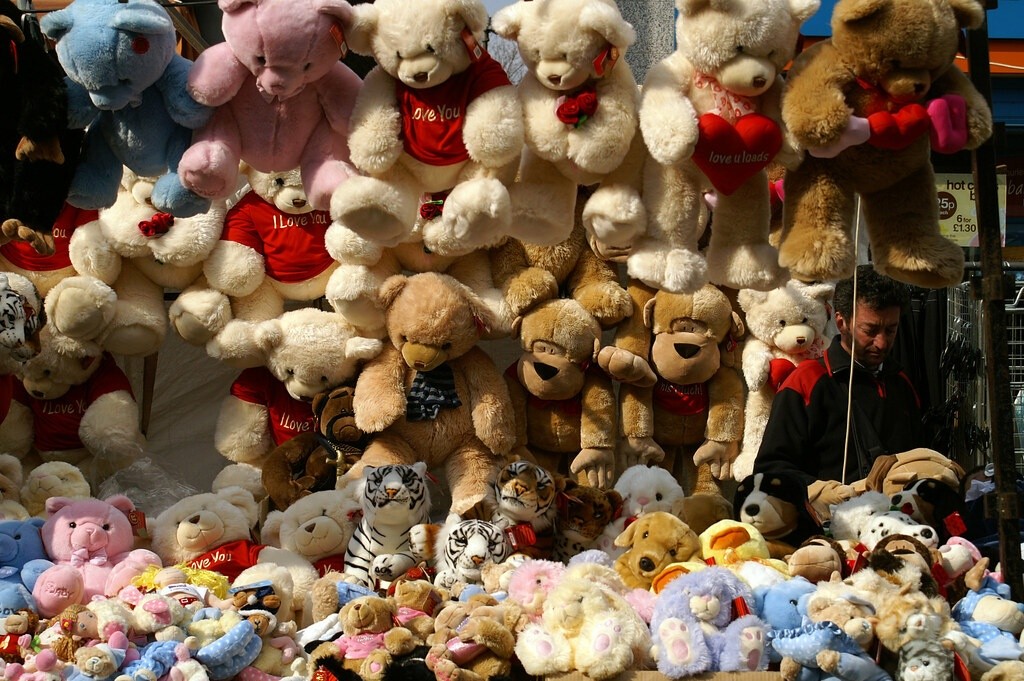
[0,1,1024,681]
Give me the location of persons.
[754,265,925,512]
